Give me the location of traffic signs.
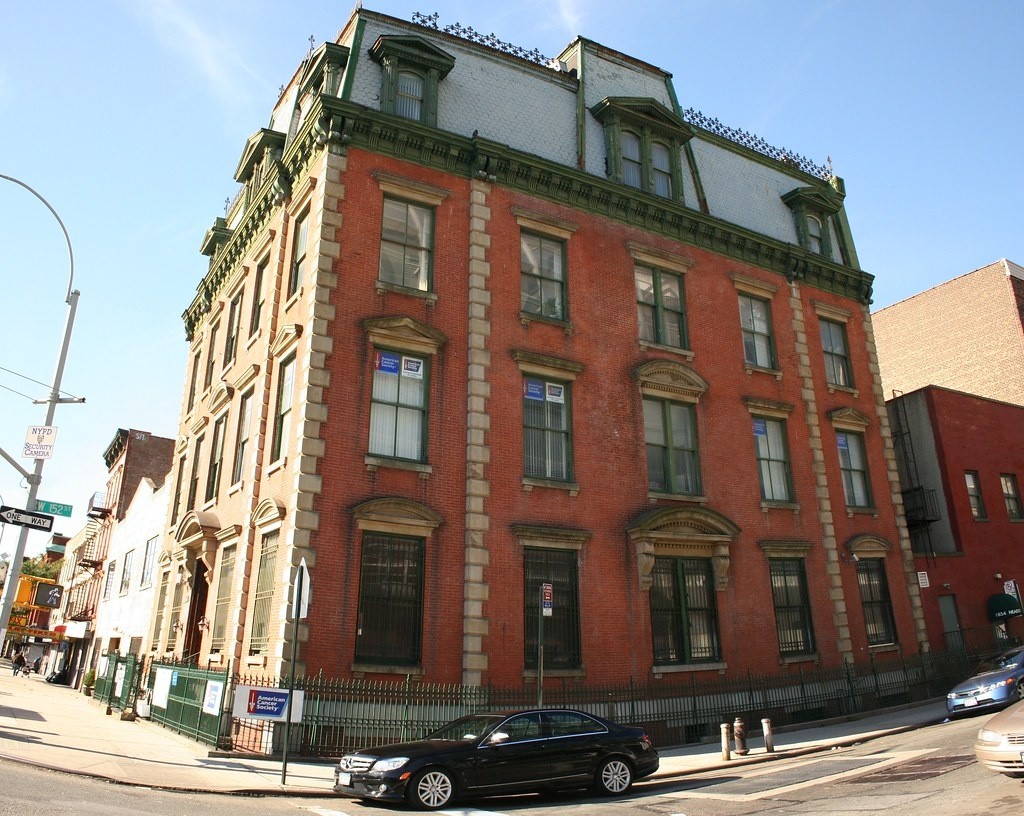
[0,505,54,533]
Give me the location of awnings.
[988,593,1022,622]
[64,622,93,638]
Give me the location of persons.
[12,651,25,676]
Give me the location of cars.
[333,706,660,811]
[946,645,1024,718]
[974,697,1024,779]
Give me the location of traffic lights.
[32,583,64,610]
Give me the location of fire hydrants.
[731,715,751,756]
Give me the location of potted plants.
[89,679,97,697]
[82,667,95,696]
